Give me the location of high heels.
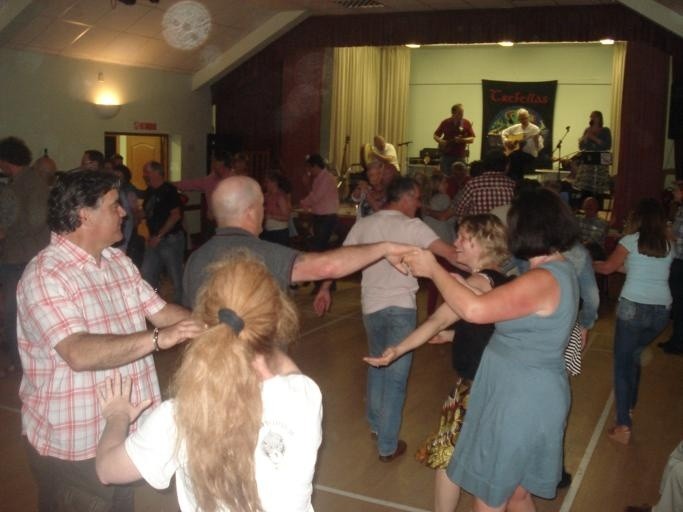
[607,426,631,444]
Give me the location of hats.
[305,153,327,164]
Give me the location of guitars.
[504,127,548,157]
[336,137,349,198]
[438,134,467,154]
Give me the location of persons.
[93,250,324,512]
[573,111,610,196]
[358,211,519,510]
[500,106,543,176]
[1,134,683,388]
[403,185,579,510]
[14,167,206,511]
[555,239,600,489]
[311,176,474,460]
[432,102,474,170]
[582,194,677,449]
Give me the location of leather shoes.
[378,440,407,463]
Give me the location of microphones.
[566,126,570,132]
[398,143,405,146]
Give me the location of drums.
[360,142,374,169]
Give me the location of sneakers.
[656,338,682,356]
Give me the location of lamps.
[93,87,122,120]
